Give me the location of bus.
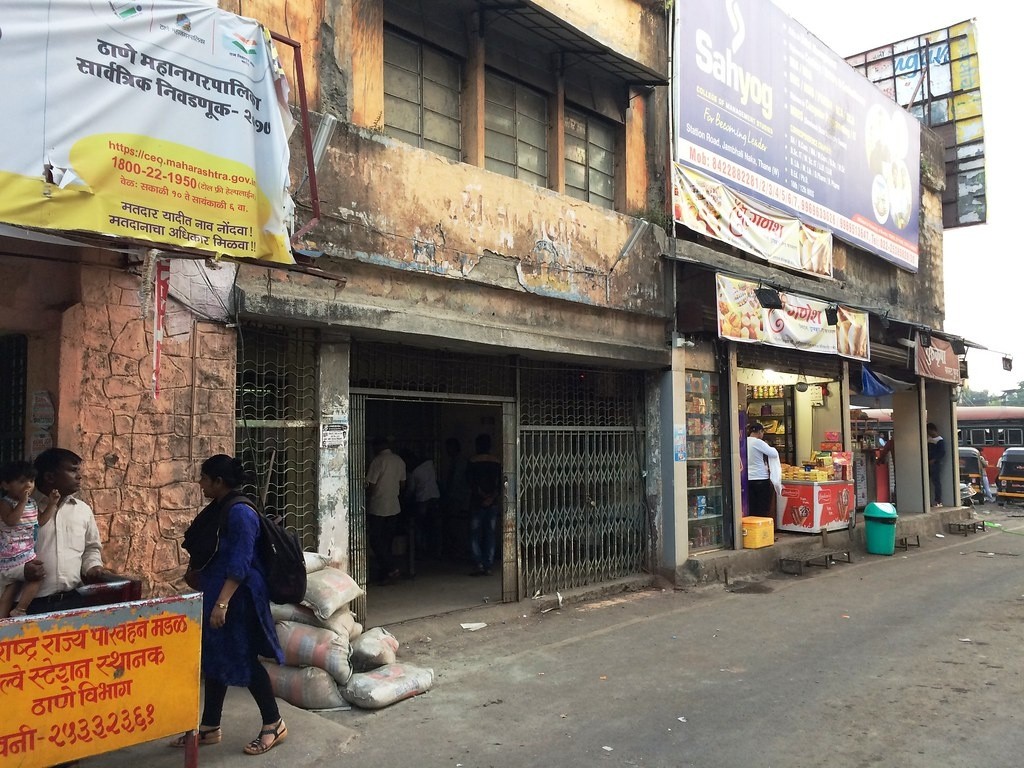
[850,406,1023,504]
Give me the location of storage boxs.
[737,382,747,411]
[782,432,854,482]
[771,405,790,416]
[742,516,774,549]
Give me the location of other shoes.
[774,536,778,541]
[9,607,28,616]
[484,566,495,577]
[932,502,943,508]
[475,562,484,576]
[372,577,399,586]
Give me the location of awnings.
[463,0,670,101]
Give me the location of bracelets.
[216,603,228,610]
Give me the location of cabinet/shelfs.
[685,391,725,554]
[747,384,796,467]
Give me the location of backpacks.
[213,496,308,606]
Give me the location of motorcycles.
[994,447,1024,507]
[959,445,991,506]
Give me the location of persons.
[364,435,407,585]
[927,423,945,508]
[169,454,288,755]
[877,430,896,508]
[464,434,502,576]
[746,423,779,541]
[0,460,61,620]
[976,447,996,503]
[958,430,962,440]
[0,448,116,616]
[402,437,470,560]
[875,431,887,447]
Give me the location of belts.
[30,589,78,602]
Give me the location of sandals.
[242,719,288,755]
[170,725,222,747]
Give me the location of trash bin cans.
[862,501,898,556]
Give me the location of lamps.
[985,348,1013,371]
[752,282,784,308]
[950,337,966,355]
[824,304,839,326]
[919,327,933,348]
